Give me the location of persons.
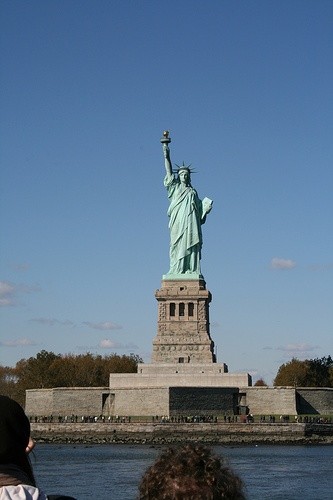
[152,414,217,423]
[0,396,47,500]
[224,414,331,423]
[161,130,213,280]
[30,413,131,423]
[139,444,245,500]
